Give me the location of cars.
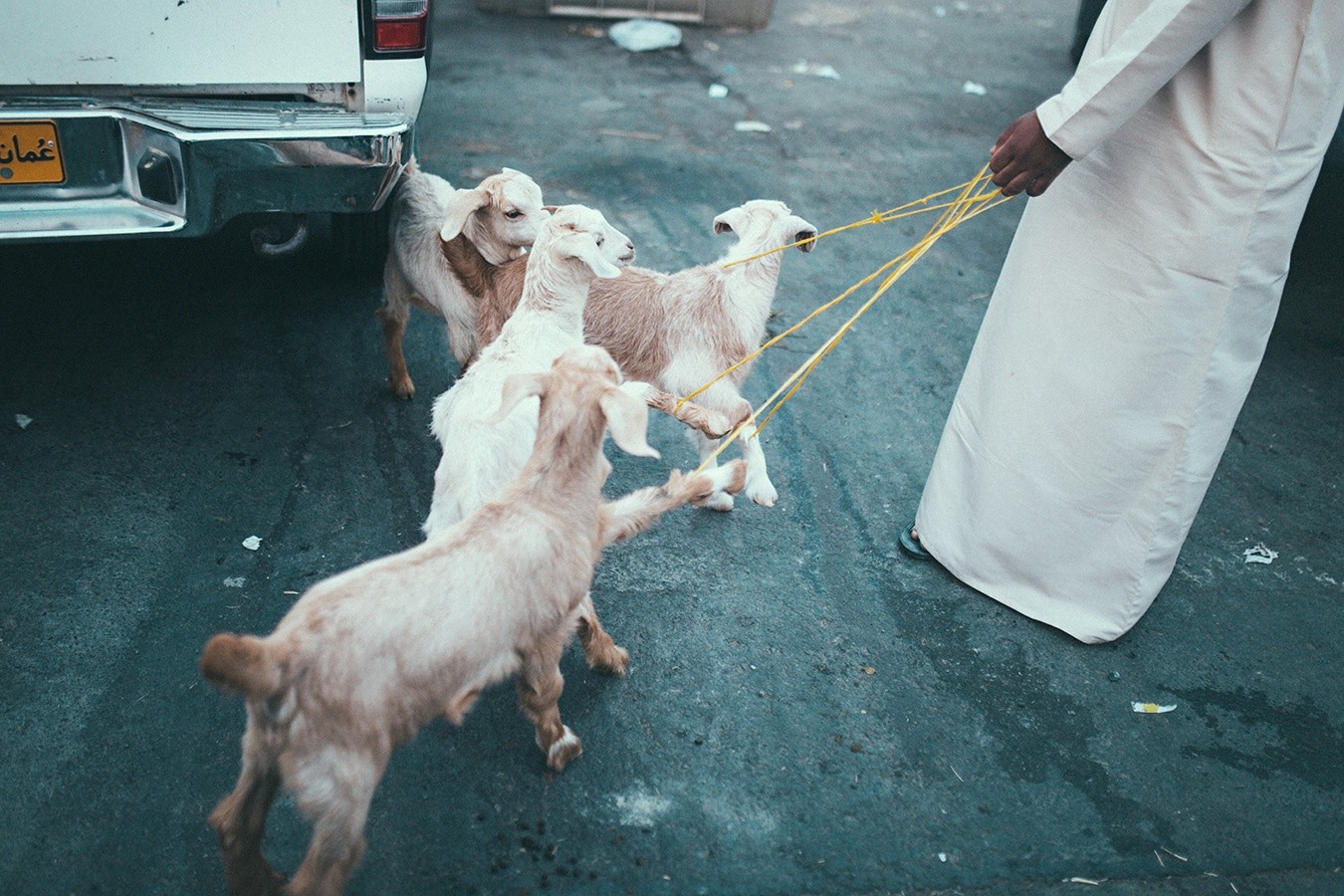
[0,1,438,286]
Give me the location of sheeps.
[422,204,633,677]
[485,197,819,514]
[380,151,553,403]
[198,344,749,896]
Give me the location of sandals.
[900,521,933,560]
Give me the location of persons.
[899,0,1344,644]
